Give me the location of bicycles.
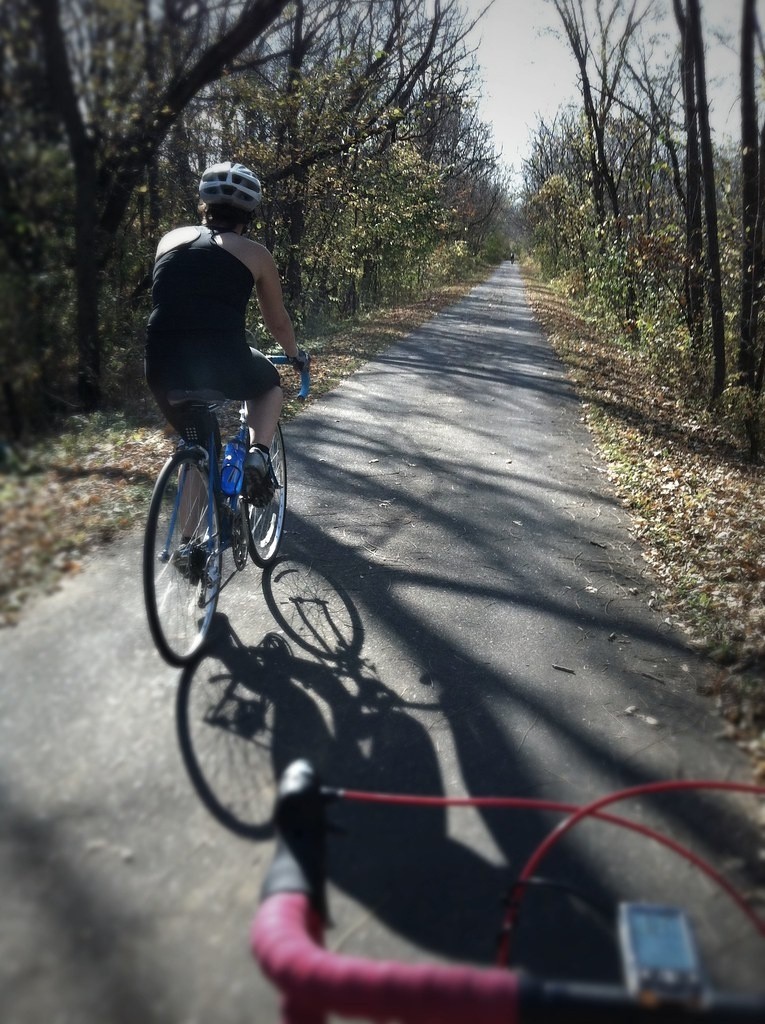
[143,354,310,668]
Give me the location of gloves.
[286,346,311,372]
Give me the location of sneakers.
[243,447,275,507]
[173,543,191,570]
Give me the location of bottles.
[220,436,247,495]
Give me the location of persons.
[144,160,303,586]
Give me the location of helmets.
[199,161,262,213]
